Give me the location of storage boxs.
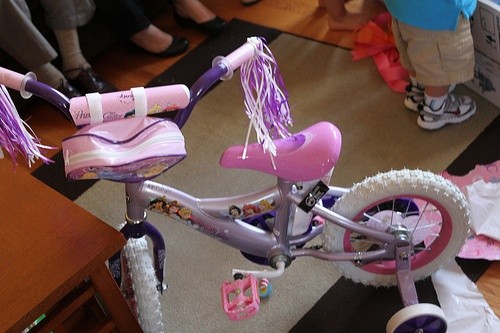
[462,0,500,103]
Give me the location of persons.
[381,0,478,131]
[93,0,232,58]
[0,0,122,121]
[316,0,374,32]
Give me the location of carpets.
[31,16,500,333]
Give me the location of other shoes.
[52,66,119,99]
[172,4,227,34]
[403,84,477,130]
[128,31,190,58]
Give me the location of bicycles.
[0,37,472,333]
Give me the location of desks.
[0,151,138,333]
